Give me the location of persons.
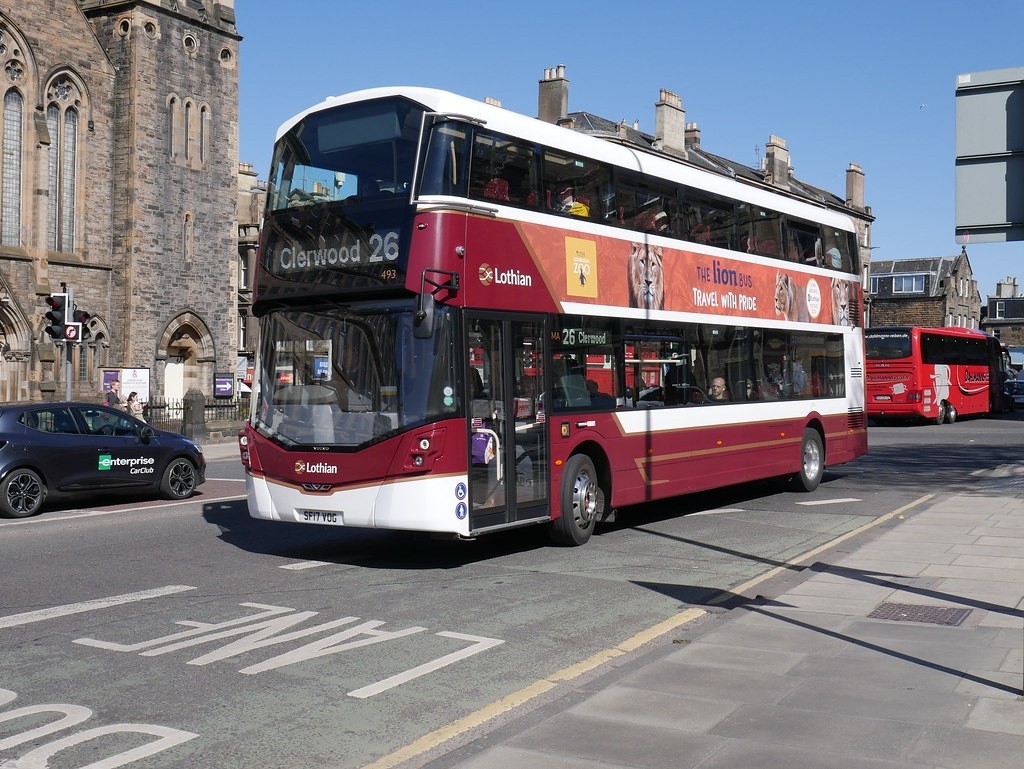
[127,392,147,424]
[703,377,730,405]
[586,380,616,406]
[747,379,753,401]
[105,379,127,427]
[553,184,590,218]
[769,356,807,400]
[825,241,842,270]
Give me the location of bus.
[237,85,869,546]
[469,340,667,398]
[864,327,1011,426]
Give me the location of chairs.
[609,206,625,226]
[634,209,656,230]
[891,350,904,359]
[759,382,781,400]
[52,408,78,434]
[734,380,748,402]
[27,412,39,429]
[484,177,509,202]
[527,189,552,211]
[572,196,591,219]
[867,350,879,358]
[670,215,851,274]
[801,373,824,397]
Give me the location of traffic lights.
[74,310,90,340]
[45,293,82,342]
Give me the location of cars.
[1002,364,1024,413]
[0,401,207,518]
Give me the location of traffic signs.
[215,378,233,396]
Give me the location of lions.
[831,277,852,326]
[628,242,664,310]
[773,268,810,323]
